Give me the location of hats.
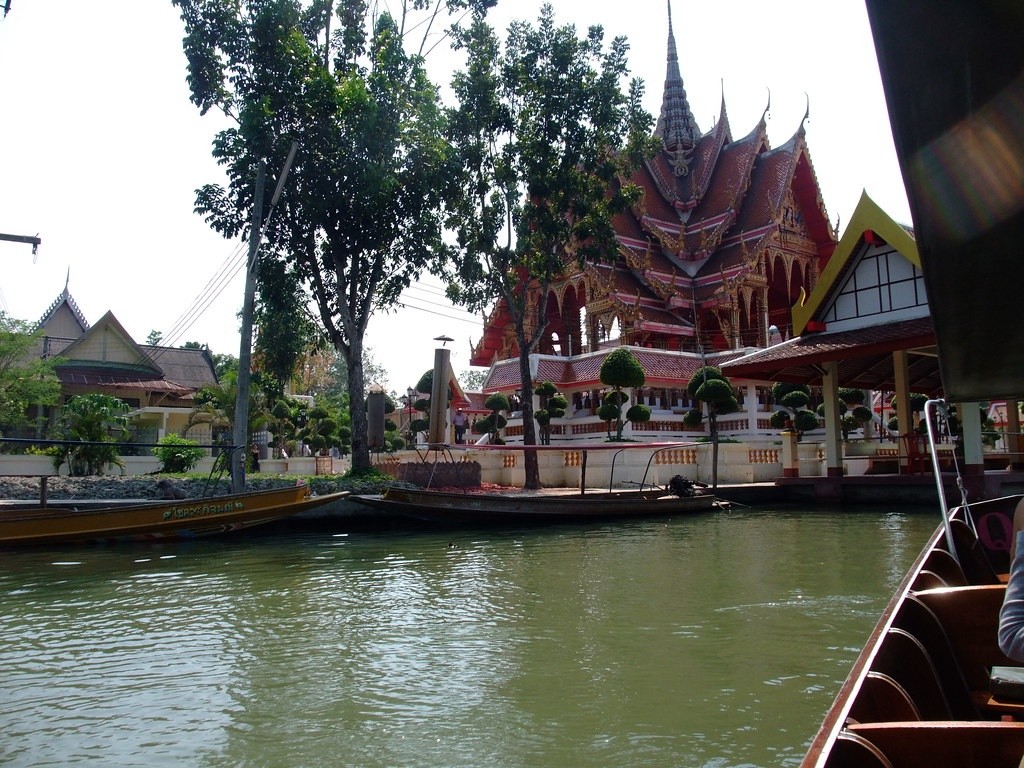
[458,408,462,411]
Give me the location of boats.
[0,434,352,551]
[348,432,728,523]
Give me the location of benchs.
[846,720,1024,768]
[918,583,1024,716]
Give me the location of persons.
[997,497,1024,663]
[314,445,342,457]
[249,445,260,471]
[453,408,465,443]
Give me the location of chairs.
[903,432,927,476]
